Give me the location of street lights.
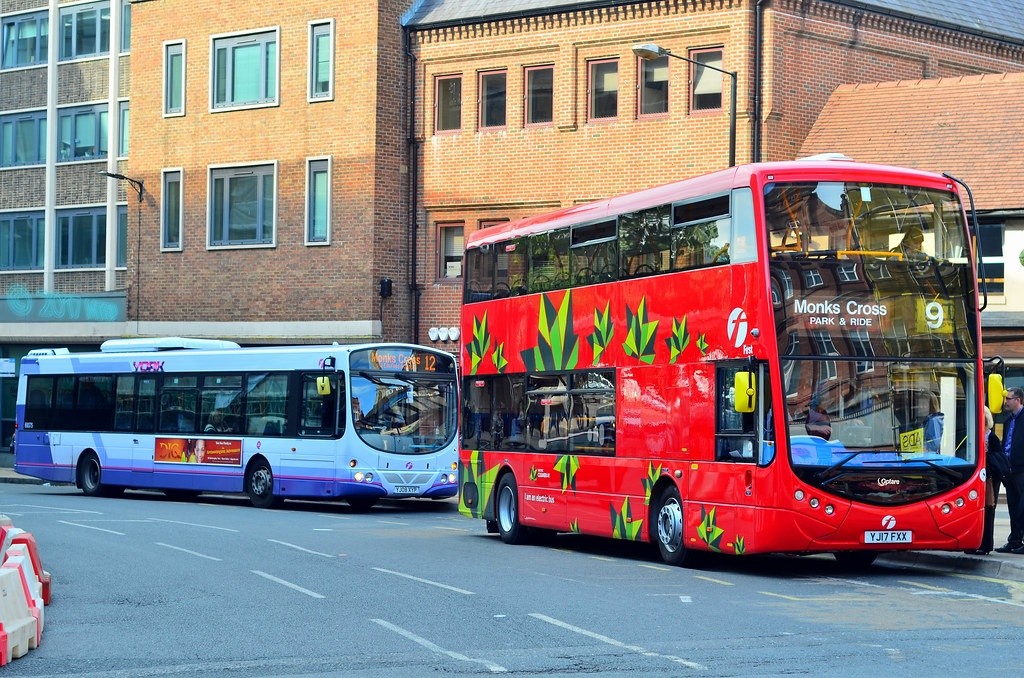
[632,45,737,166]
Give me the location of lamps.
[98,171,145,200]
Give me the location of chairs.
[469,263,655,302]
[29,389,285,436]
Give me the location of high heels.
[964,549,989,555]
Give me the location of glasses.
[1005,396,1021,400]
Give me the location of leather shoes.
[994,542,1024,553]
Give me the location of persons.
[994,387,1024,554]
[976,405,1002,555]
[917,390,945,455]
[889,226,924,253]
[193,439,207,463]
[203,411,233,432]
[766,382,865,448]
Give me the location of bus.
[456,149,1007,569]
[13,336,462,511]
